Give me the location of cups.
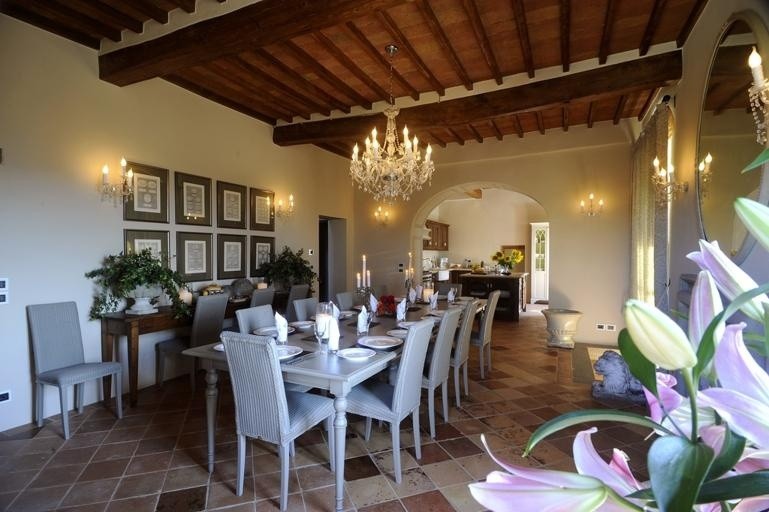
[428,294,437,312]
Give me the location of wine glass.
[313,322,325,354]
[451,287,457,303]
[362,310,375,336]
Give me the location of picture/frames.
[250,236,275,277]
[123,161,170,224]
[217,180,247,229]
[217,233,247,279]
[175,171,212,226]
[124,229,170,271]
[176,231,213,282]
[250,187,275,231]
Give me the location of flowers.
[467,146,769,512]
[492,249,523,272]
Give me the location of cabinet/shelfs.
[423,220,450,251]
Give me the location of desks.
[96,291,315,408]
[460,272,529,322]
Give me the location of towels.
[430,291,438,308]
[328,301,340,321]
[328,319,340,350]
[448,290,454,301]
[275,311,288,341]
[397,298,406,320]
[358,305,368,333]
[370,293,377,312]
[409,288,416,304]
[416,285,422,299]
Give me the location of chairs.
[334,318,434,483]
[235,304,276,334]
[26,302,123,440]
[220,331,337,511]
[222,288,273,333]
[293,298,317,321]
[466,277,488,299]
[279,284,309,323]
[425,298,478,408]
[489,277,516,321]
[155,294,228,400]
[336,292,353,311]
[377,308,462,441]
[454,291,501,380]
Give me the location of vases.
[501,264,511,274]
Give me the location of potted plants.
[85,247,194,321]
[260,246,320,292]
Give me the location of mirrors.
[695,9,769,266]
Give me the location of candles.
[279,200,282,212]
[413,134,418,153]
[748,46,764,86]
[659,168,667,190]
[599,200,603,212]
[288,194,293,212]
[403,124,408,144]
[372,127,378,158]
[698,153,712,175]
[120,157,127,176]
[102,164,109,184]
[589,193,593,211]
[375,212,378,221]
[352,142,358,162]
[378,206,382,216]
[581,200,584,213]
[653,156,659,175]
[127,168,133,187]
[405,140,411,155]
[425,143,432,162]
[362,151,366,160]
[417,151,421,161]
[385,211,388,222]
[365,137,370,154]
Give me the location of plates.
[288,321,314,329]
[211,343,224,351]
[339,311,357,315]
[395,321,415,329]
[310,314,346,320]
[275,345,303,361]
[252,325,295,336]
[334,347,376,361]
[356,336,403,349]
[386,330,408,338]
[352,305,369,310]
